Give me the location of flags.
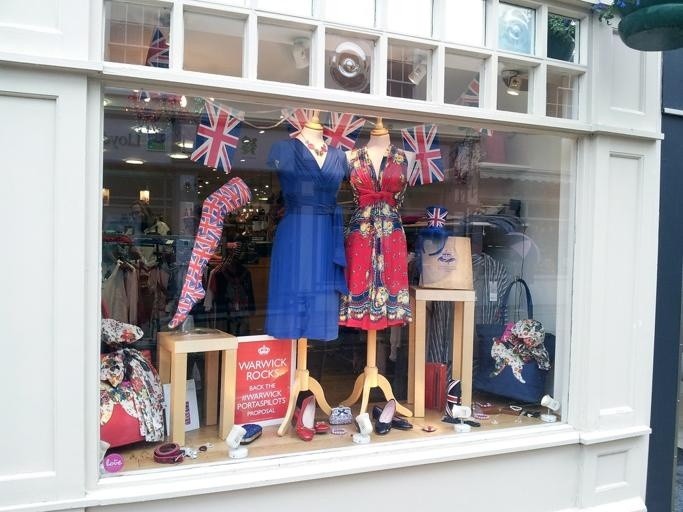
[400,123,448,187]
[188,98,245,175]
[282,108,314,138]
[461,71,479,107]
[135,15,169,102]
[321,111,366,153]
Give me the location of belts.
[153,443,183,464]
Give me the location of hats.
[421,206,451,236]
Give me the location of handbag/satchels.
[473,279,556,405]
[329,405,352,424]
[418,235,474,290]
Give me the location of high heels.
[292,395,331,441]
[373,400,413,436]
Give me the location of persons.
[267,121,349,342]
[336,130,416,332]
[128,199,153,223]
[166,176,255,329]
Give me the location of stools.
[157,328,237,447]
[408,285,477,419]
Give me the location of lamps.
[290,36,523,96]
